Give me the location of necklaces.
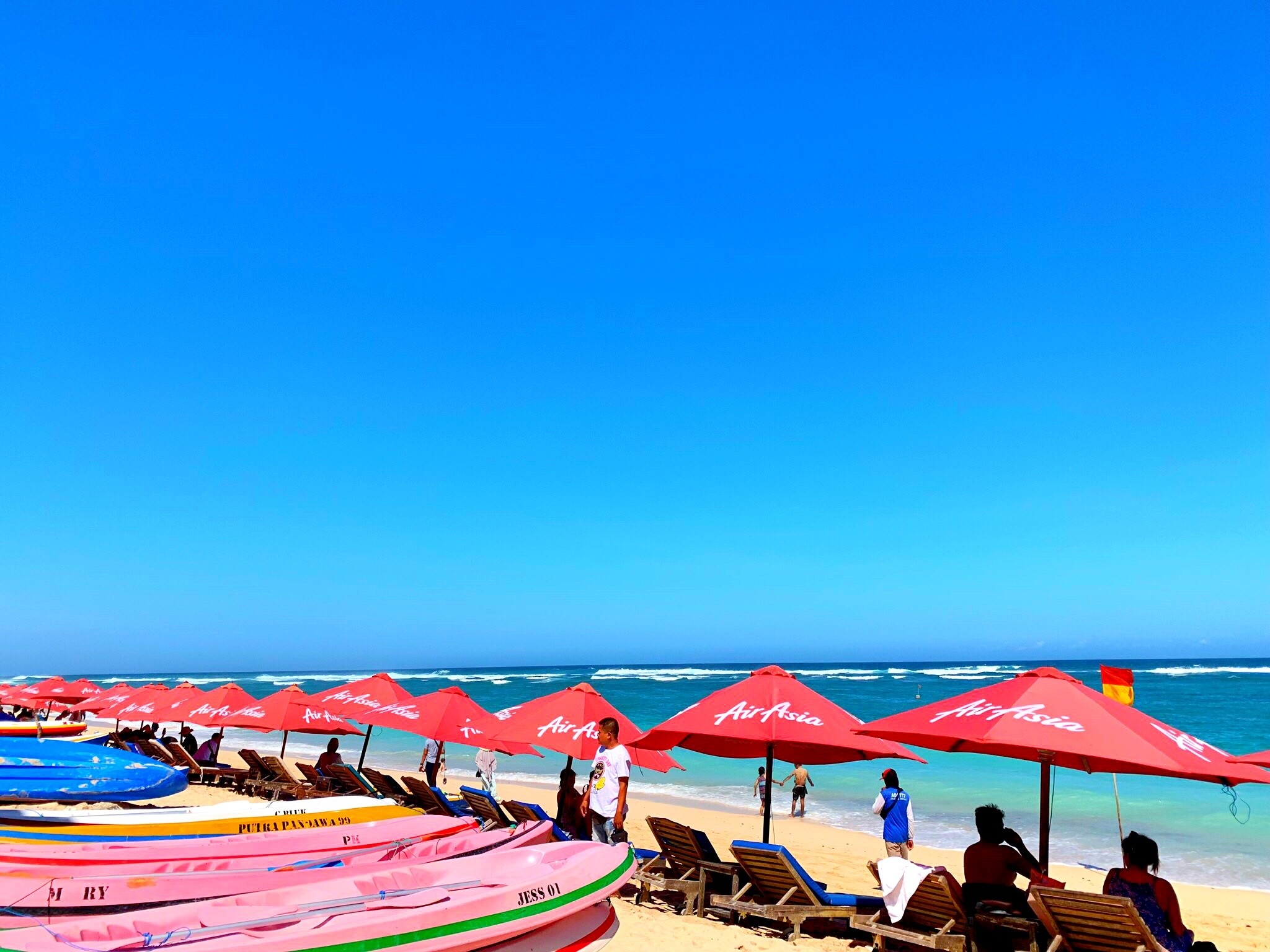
[963,804,1048,952]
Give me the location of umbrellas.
[0,673,274,727]
[850,666,1270,877]
[1226,750,1270,768]
[625,664,927,846]
[204,682,368,760]
[491,681,686,774]
[339,684,546,791]
[287,671,416,776]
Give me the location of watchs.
[441,759,445,762]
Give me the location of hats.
[879,768,896,781]
[212,733,224,739]
[329,738,338,745]
[178,726,194,734]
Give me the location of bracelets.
[420,763,423,766]
[583,799,589,801]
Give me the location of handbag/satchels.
[208,739,216,760]
[879,788,904,821]
[610,829,628,843]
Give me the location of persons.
[418,738,447,787]
[192,732,221,784]
[872,768,914,860]
[555,766,597,841]
[580,716,630,900]
[475,748,501,801]
[779,763,814,817]
[753,766,784,816]
[1103,830,1219,952]
[319,736,344,792]
[0,704,198,761]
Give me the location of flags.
[1099,664,1135,706]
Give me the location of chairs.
[111,735,1171,952]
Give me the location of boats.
[3,794,643,951]
[0,718,88,736]
[42,730,110,746]
[0,735,190,804]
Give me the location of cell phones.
[164,728,165,731]
